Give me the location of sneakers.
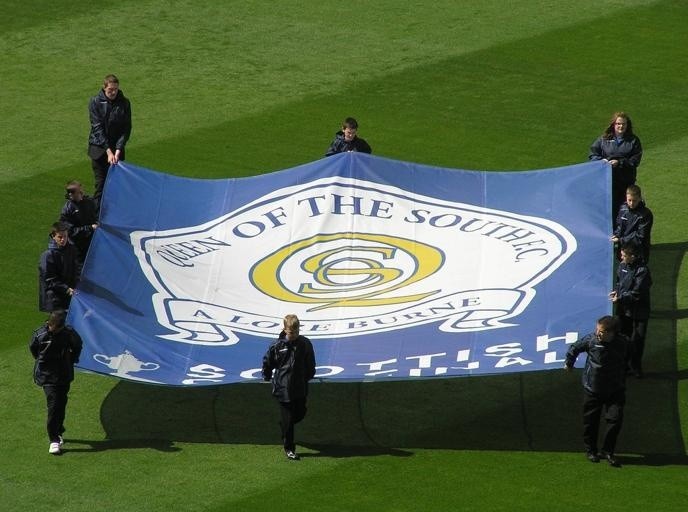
[285,450,300,460]
[600,447,622,468]
[633,368,645,379]
[48,434,65,454]
[586,451,601,462]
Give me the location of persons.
[612,184,653,265]
[28,310,83,455]
[588,111,643,234]
[261,314,316,460]
[563,315,636,466]
[87,74,132,207]
[608,239,653,379]
[325,116,372,154]
[60,180,99,264]
[38,221,80,315]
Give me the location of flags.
[65,148,614,390]
